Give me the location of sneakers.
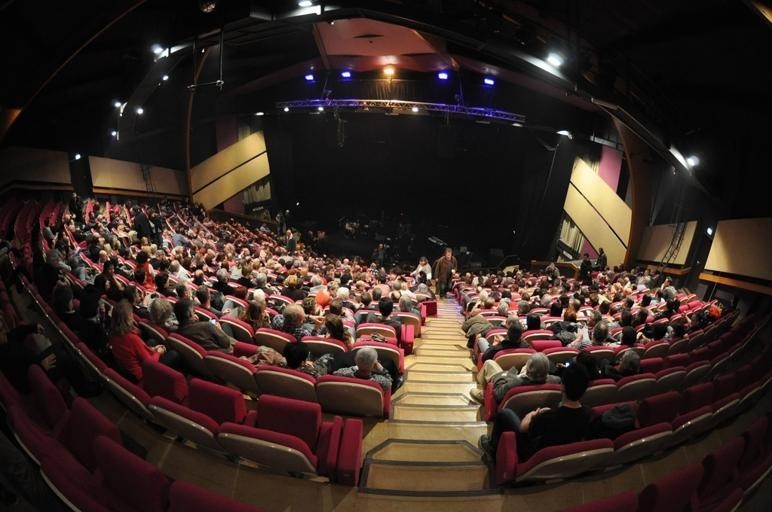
[478,435,494,463]
[469,388,485,406]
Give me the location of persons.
[433,248,457,298]
[1,186,432,399]
[452,247,731,463]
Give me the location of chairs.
[451,270,771,512]
[1,191,436,512]
